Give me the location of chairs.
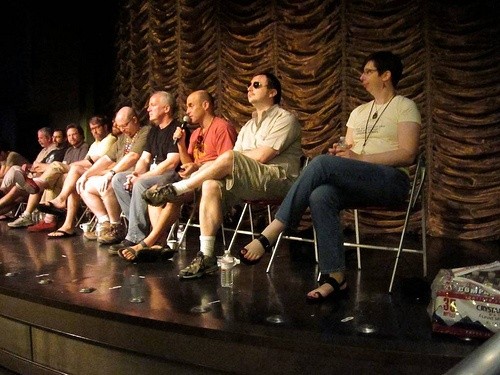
[13,132,434,293]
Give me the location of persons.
[119,90,236,262]
[238,50,422,304]
[0,116,123,239]
[76,106,152,244]
[140,71,302,278]
[108,91,191,253]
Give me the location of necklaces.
[363,94,396,146]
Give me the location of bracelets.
[110,170,116,175]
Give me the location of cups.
[79,222,90,233]
[167,240,176,250]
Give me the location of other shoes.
[15,170,40,194]
[27,220,58,232]
[7,215,34,227]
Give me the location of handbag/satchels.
[427,260,500,338]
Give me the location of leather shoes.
[108,237,135,254]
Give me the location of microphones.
[173,116,189,144]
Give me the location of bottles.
[177,224,186,250]
[220,250,234,288]
[217,257,240,267]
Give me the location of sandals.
[238,233,276,265]
[308,277,348,299]
[135,241,175,261]
[117,242,148,262]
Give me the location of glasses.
[247,82,269,89]
[51,136,65,140]
[117,118,133,129]
[90,123,103,133]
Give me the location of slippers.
[35,202,66,216]
[0,214,19,222]
[48,228,77,238]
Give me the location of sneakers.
[97,223,125,244]
[176,251,218,278]
[84,221,110,239]
[141,184,177,209]
